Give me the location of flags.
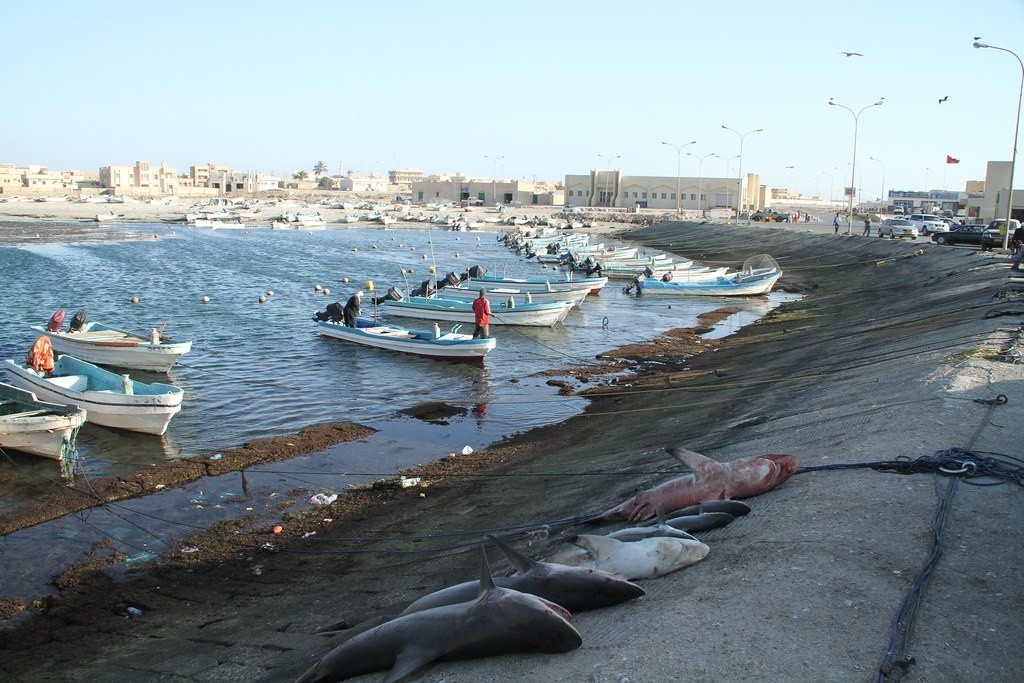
[947,154,960,163]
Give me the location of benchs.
[6,409,45,417]
[65,331,146,342]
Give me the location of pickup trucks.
[750,206,792,223]
[461,197,485,207]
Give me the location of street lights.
[686,152,716,211]
[835,167,845,211]
[722,125,764,223]
[869,157,885,225]
[598,155,622,207]
[823,172,833,205]
[485,156,504,200]
[662,141,696,209]
[715,155,741,208]
[848,163,861,212]
[972,41,1024,250]
[829,100,884,235]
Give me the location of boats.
[0,383,87,463]
[29,307,192,374]
[0,192,141,206]
[96,209,119,223]
[383,263,576,328]
[311,287,496,363]
[145,172,783,320]
[4,352,185,438]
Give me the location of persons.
[524,214,527,220]
[756,208,808,223]
[1012,222,1024,246]
[643,265,653,277]
[833,213,841,233]
[343,290,364,328]
[863,214,871,237]
[663,270,673,282]
[1010,240,1024,271]
[472,287,495,339]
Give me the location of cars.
[509,200,522,206]
[931,223,988,246]
[893,206,905,215]
[893,206,967,231]
[878,218,919,241]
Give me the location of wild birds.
[830,97,834,101]
[786,166,795,168]
[939,96,949,103]
[974,37,981,41]
[880,97,884,100]
[839,51,863,57]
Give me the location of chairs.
[995,222,1000,228]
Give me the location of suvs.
[980,218,1022,251]
[907,213,951,236]
